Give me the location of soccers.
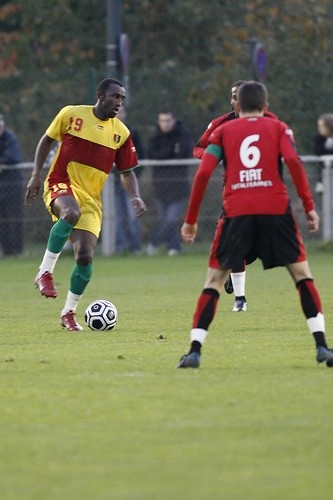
[83,300,115,330]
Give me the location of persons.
[25,78,147,331]
[314,113,333,196]
[114,103,143,254]
[178,81,333,369]
[145,110,194,256]
[193,80,280,314]
[0,115,26,256]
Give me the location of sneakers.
[317,345,333,366]
[233,299,246,311]
[34,271,57,299]
[60,311,83,331]
[176,352,200,368]
[224,273,234,294]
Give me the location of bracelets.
[131,197,142,202]
